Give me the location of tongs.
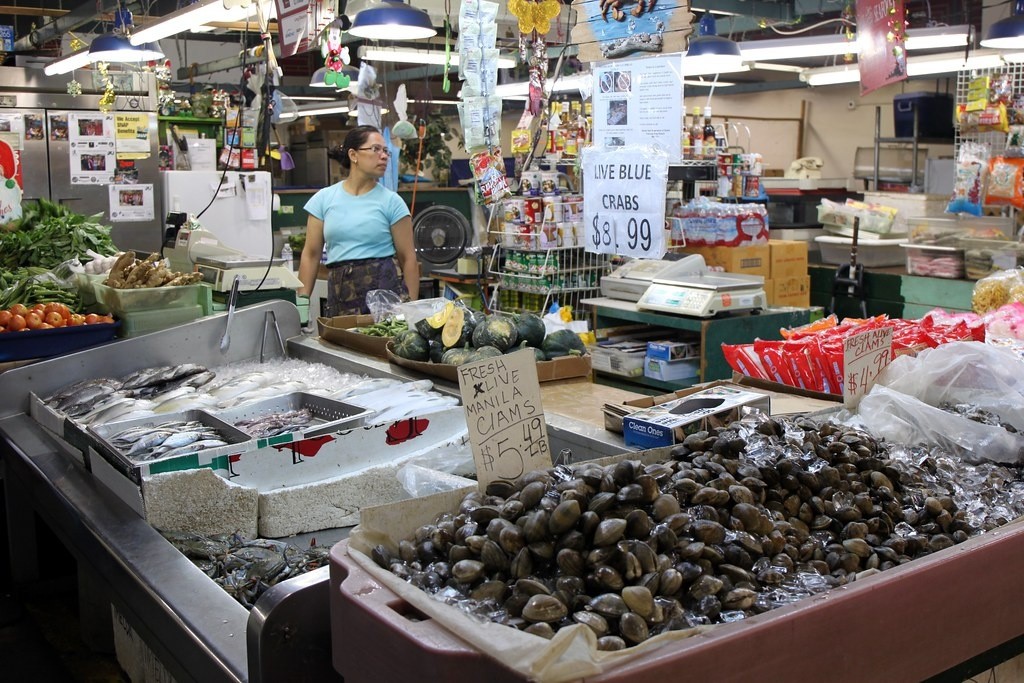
[259,310,287,363]
[220,274,240,356]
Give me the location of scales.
[636,273,767,319]
[195,254,304,292]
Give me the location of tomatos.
[0,302,115,332]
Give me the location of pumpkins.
[393,304,586,366]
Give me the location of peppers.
[363,317,408,338]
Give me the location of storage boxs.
[319,314,700,382]
[622,385,772,452]
[673,177,1024,308]
[0,277,204,361]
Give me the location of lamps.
[357,45,518,68]
[688,10,741,59]
[43,46,89,76]
[980,0,1024,49]
[89,5,165,62]
[348,1,437,39]
[309,63,359,87]
[280,101,356,118]
[739,26,976,61]
[802,47,1024,86]
[141,0,250,58]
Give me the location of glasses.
[357,144,392,156]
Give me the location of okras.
[0,278,82,314]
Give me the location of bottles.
[670,105,770,251]
[545,94,595,161]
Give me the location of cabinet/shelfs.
[158,115,225,148]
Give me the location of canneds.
[717,153,763,199]
[493,169,598,310]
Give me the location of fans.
[405,205,474,275]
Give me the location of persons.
[297,125,420,333]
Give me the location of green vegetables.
[0,196,120,268]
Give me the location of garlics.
[85,256,121,275]
